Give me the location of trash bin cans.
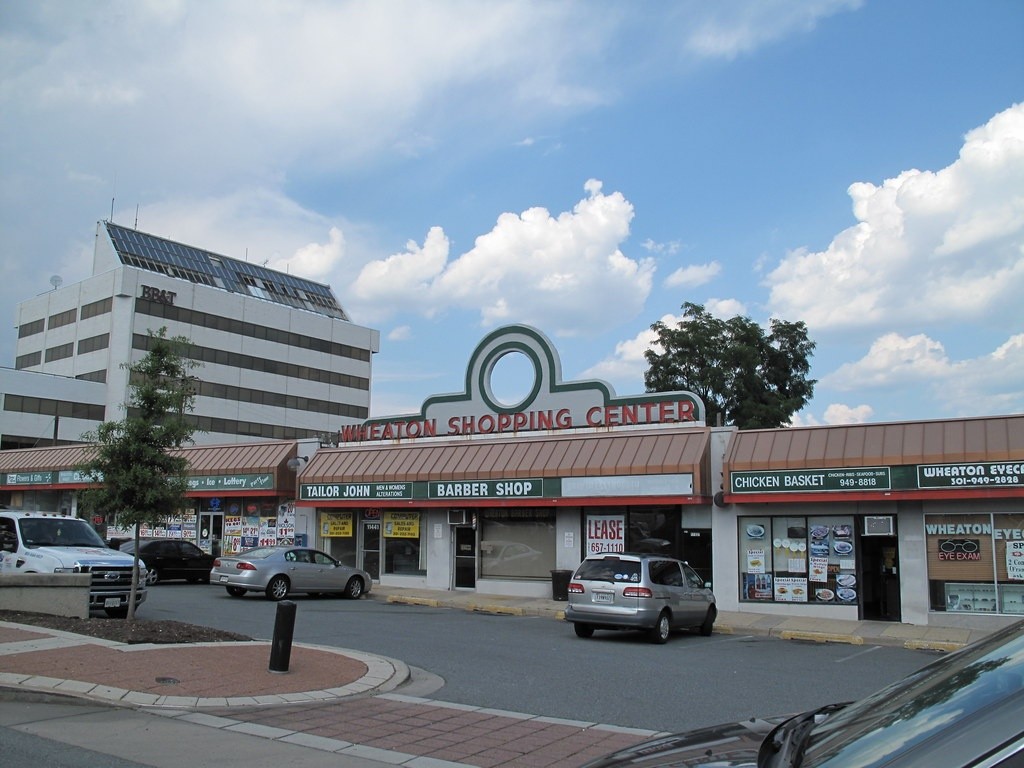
[111,538,131,551]
[550,570,574,601]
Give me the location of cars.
[210,544,372,601]
[564,550,717,643]
[117,537,223,586]
[580,617,1024,767]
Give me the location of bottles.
[767,575,771,589]
[756,575,760,589]
[762,576,766,589]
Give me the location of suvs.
[1,507,149,619]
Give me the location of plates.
[833,542,852,553]
[781,539,790,548]
[816,589,835,600]
[748,559,763,568]
[798,543,807,552]
[777,586,805,596]
[773,538,781,548]
[836,589,856,600]
[811,567,825,576]
[812,528,829,538]
[746,524,764,536]
[836,575,856,586]
[789,543,799,552]
